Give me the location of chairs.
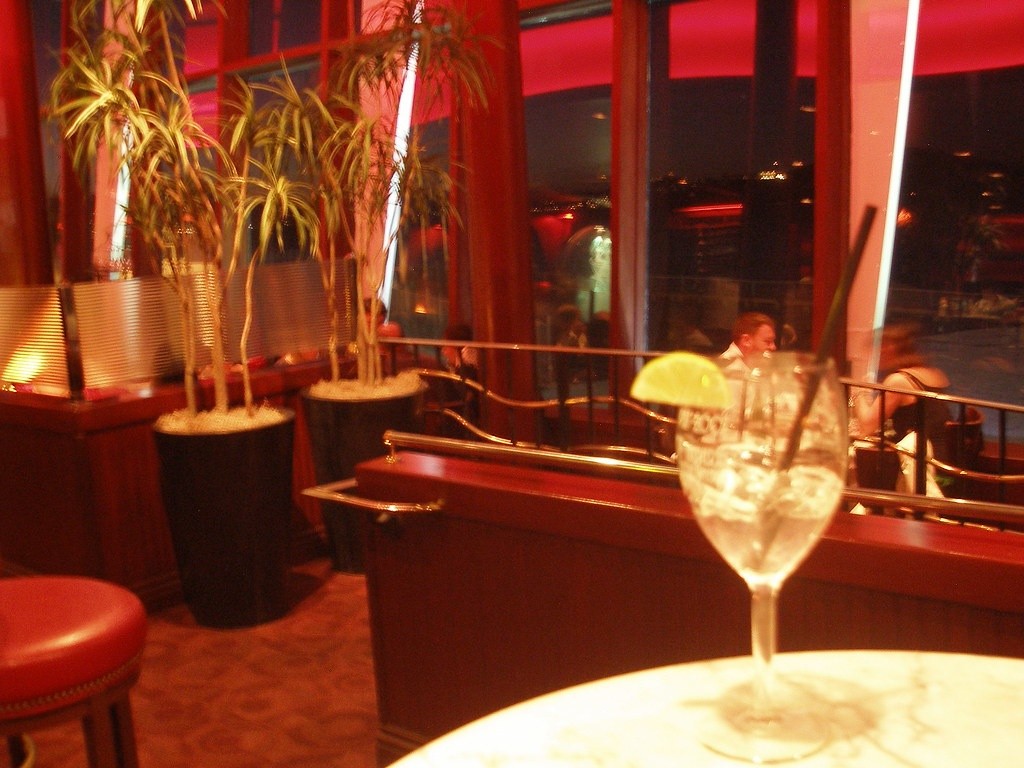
[941,404,985,501]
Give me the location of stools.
[1,577,150,767]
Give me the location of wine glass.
[676,352,847,758]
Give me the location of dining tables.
[392,648,1024,768]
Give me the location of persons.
[849,323,957,515]
[718,312,778,361]
[448,326,481,400]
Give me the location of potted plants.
[226,1,506,573]
[43,1,324,628]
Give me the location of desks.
[849,419,900,491]
